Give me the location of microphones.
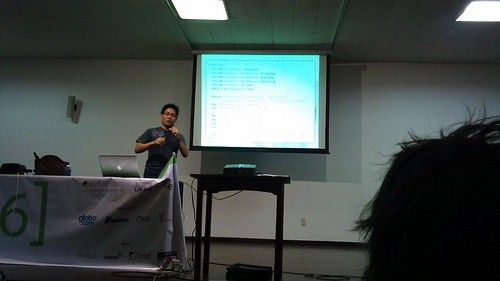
[170,124,176,136]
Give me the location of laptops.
[98,155,141,178]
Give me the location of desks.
[0,174,184,281]
[190,174,291,281]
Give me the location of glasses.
[162,112,178,118]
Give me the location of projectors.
[224,163,257,176]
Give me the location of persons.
[348,116,500,281]
[134,103,189,178]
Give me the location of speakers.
[65,96,75,119]
